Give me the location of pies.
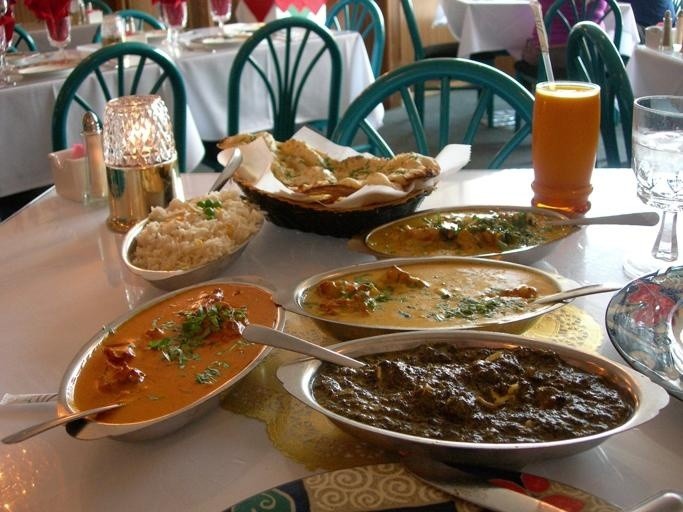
[216,131,440,204]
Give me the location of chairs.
[0,0,683,188]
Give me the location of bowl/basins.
[228,170,426,237]
[363,205,587,266]
[121,195,264,294]
[267,258,580,345]
[46,147,91,205]
[270,327,672,469]
[56,275,284,443]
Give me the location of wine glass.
[0,0,230,87]
[629,96,683,259]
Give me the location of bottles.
[660,9,673,52]
[675,10,682,43]
[79,111,108,200]
[99,94,185,235]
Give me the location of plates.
[603,268,682,404]
[223,456,630,512]
[189,33,253,46]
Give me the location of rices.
[132,190,264,271]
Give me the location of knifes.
[397,452,569,512]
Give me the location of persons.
[630,0,675,45]
[523,0,608,80]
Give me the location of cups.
[643,26,662,49]
[530,80,602,217]
